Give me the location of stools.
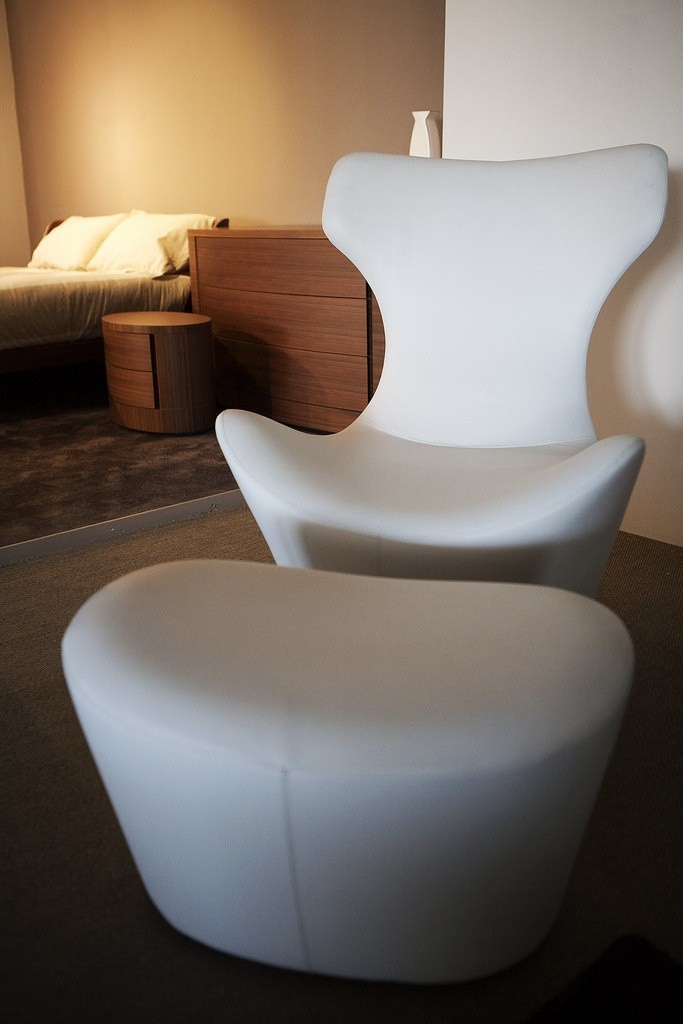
[62,559,634,984]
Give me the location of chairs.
[216,143,669,595]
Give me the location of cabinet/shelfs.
[187,225,386,433]
[101,311,213,434]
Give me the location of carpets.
[0,404,241,548]
[0,488,682,1024]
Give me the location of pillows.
[84,209,216,279]
[27,213,130,271]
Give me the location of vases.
[408,110,441,157]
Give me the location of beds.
[0,209,229,373]
